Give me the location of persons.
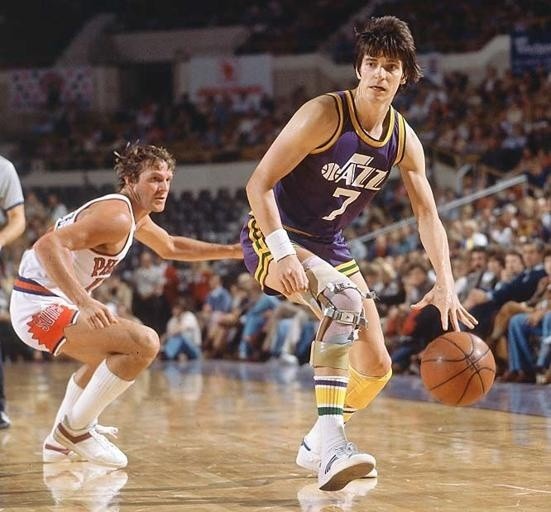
[98,251,317,365]
[0,93,303,169]
[345,187,473,374]
[23,191,44,216]
[463,190,551,384]
[0,154,26,429]
[240,17,478,492]
[44,191,66,227]
[9,142,244,466]
[400,71,550,191]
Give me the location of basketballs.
[420,333,497,408]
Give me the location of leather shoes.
[498,372,514,384]
[513,371,534,383]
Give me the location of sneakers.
[0,407,10,429]
[296,439,380,479]
[44,440,85,462]
[53,413,129,470]
[317,440,376,492]
